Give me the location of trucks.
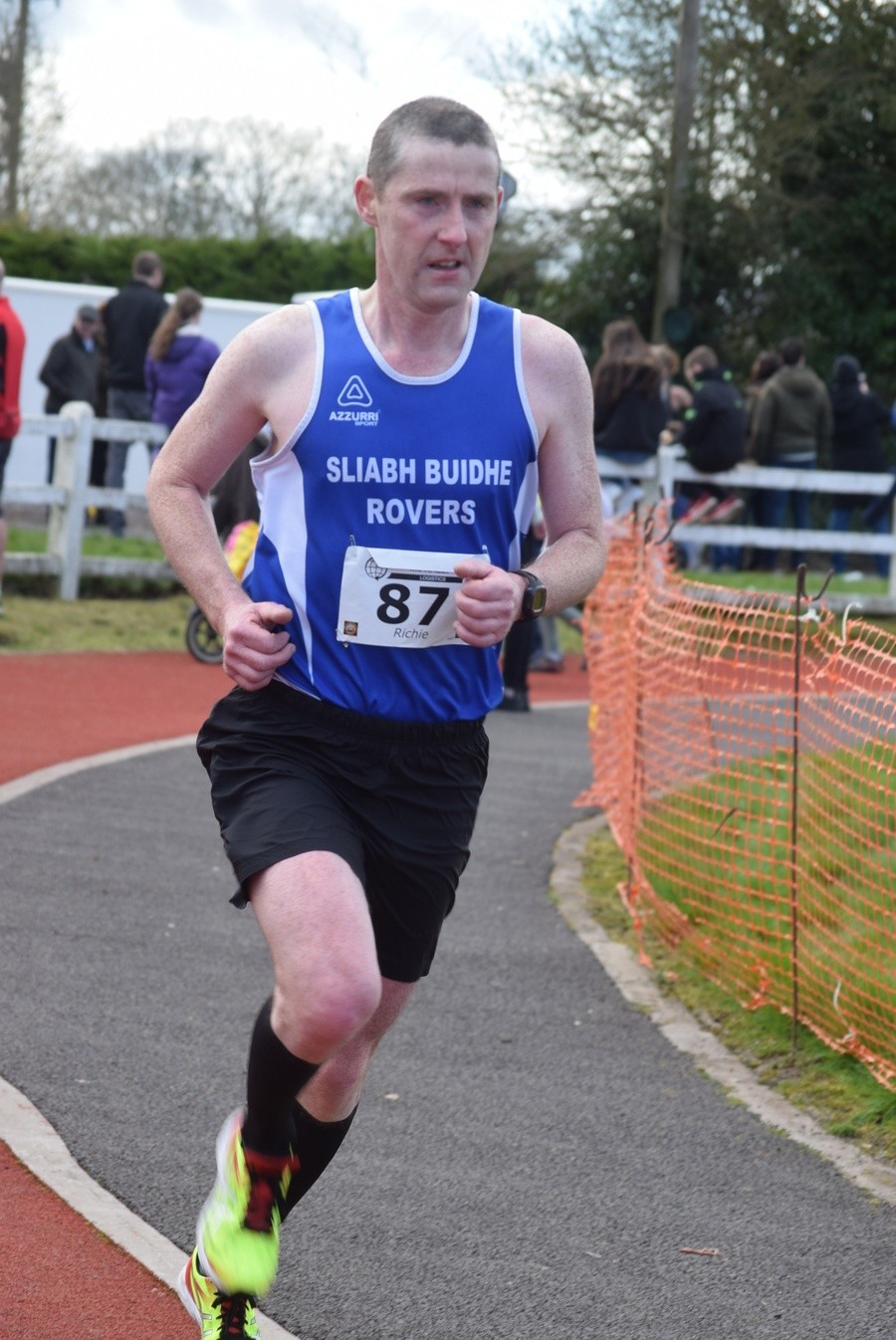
[1,277,314,522]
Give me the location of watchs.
[508,569,548,625]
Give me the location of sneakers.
[178,1246,261,1340]
[196,1108,292,1299]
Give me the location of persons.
[1,257,26,619]
[592,318,895,583]
[145,96,608,1340]
[38,251,221,539]
[497,494,547,711]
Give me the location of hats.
[78,306,97,323]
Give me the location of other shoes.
[494,689,527,710]
